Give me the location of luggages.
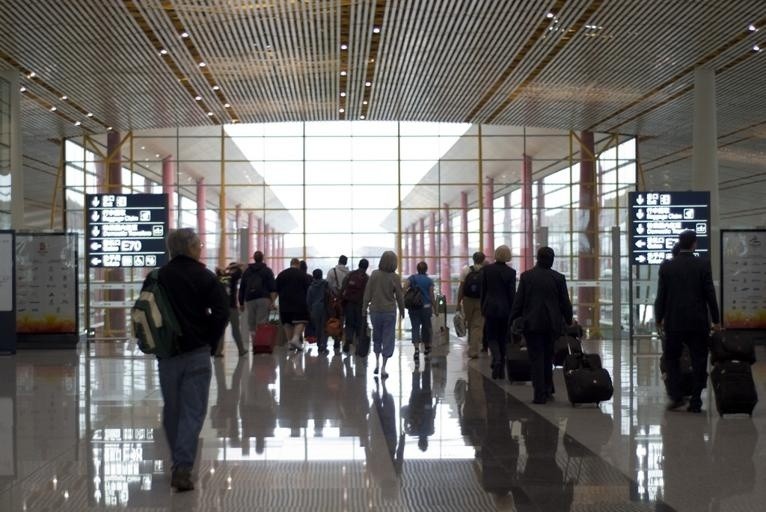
[254,308,277,354]
[563,330,614,407]
[503,333,533,384]
[709,327,758,417]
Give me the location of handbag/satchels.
[327,286,344,310]
[324,317,343,337]
[357,316,370,358]
[403,287,424,310]
[306,321,318,343]
[563,353,601,374]
[710,332,756,366]
[454,311,466,337]
[131,267,184,358]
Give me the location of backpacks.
[341,270,368,303]
[462,265,486,300]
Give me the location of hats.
[226,263,242,270]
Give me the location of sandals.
[414,348,419,360]
[424,346,432,354]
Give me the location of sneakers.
[172,479,193,491]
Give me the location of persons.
[672,243,681,259]
[362,251,406,375]
[212,354,440,501]
[404,260,437,360]
[462,354,575,510]
[139,227,229,491]
[217,250,370,354]
[455,243,573,404]
[653,232,722,412]
